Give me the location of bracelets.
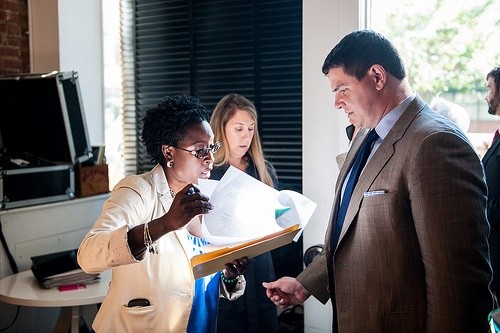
[222,272,239,283]
[143,222,160,254]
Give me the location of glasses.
[174,141,221,159]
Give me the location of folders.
[191,224,302,279]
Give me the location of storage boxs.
[79,163,111,197]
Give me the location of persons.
[77,95,251,333]
[429,95,470,133]
[263,29,500,333]
[204,93,280,333]
[481,67,500,308]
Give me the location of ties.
[328,128,380,257]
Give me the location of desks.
[0,268,112,333]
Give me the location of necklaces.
[244,157,249,167]
[170,189,178,198]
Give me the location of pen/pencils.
[189,187,194,194]
